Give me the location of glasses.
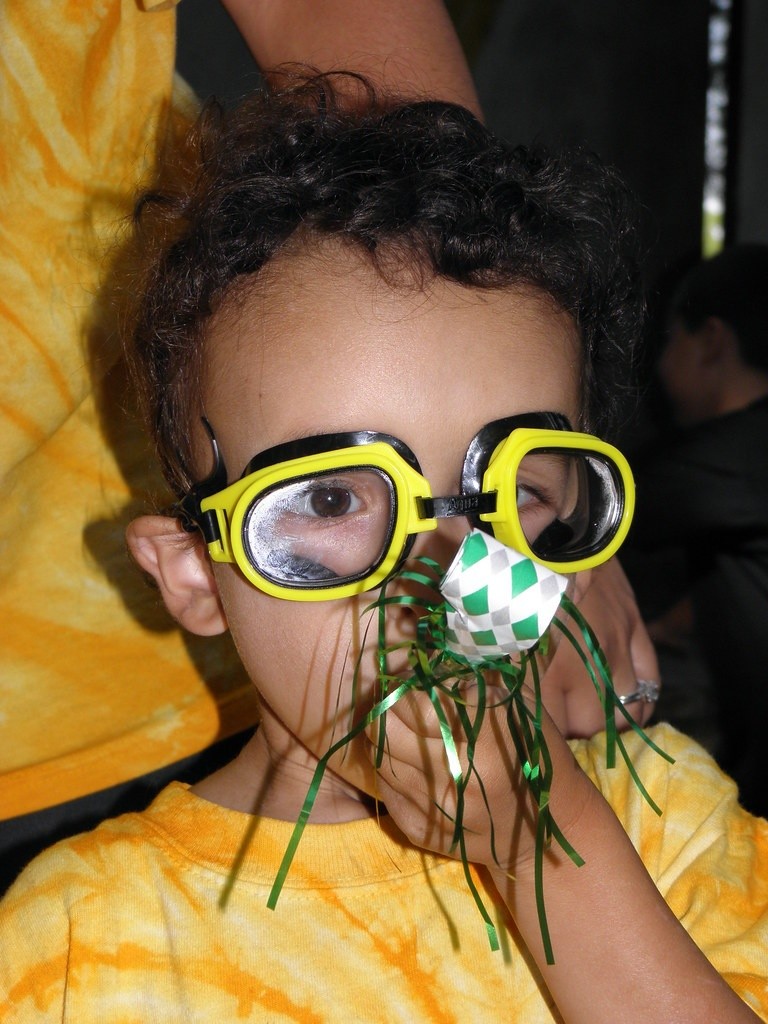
[176,412,635,601]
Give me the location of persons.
[0,69,768,1024]
[0,0,768,894]
